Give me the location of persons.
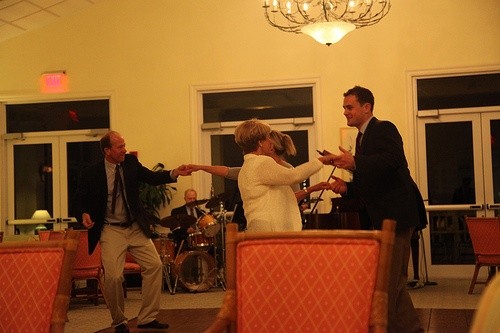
[187,131,301,192]
[331,85,428,333]
[171,188,206,252]
[235,120,342,234]
[73,131,192,333]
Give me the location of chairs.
[465,216,500,296]
[0,219,412,333]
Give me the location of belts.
[111,219,136,227]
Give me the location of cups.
[38,231,50,241]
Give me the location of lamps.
[264,0,390,45]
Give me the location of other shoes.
[137,319,169,329]
[115,323,130,333]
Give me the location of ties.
[356,132,366,211]
[111,165,120,214]
[190,207,194,216]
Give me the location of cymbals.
[185,200,207,207]
[160,214,197,227]
[205,191,233,208]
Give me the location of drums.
[197,214,221,238]
[172,251,217,292]
[153,238,174,263]
[189,233,215,250]
